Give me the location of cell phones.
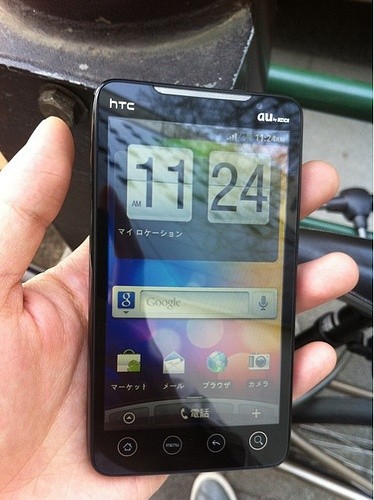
[87,76,311,476]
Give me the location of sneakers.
[187,471,237,500]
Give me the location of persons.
[0,116,359,499]
